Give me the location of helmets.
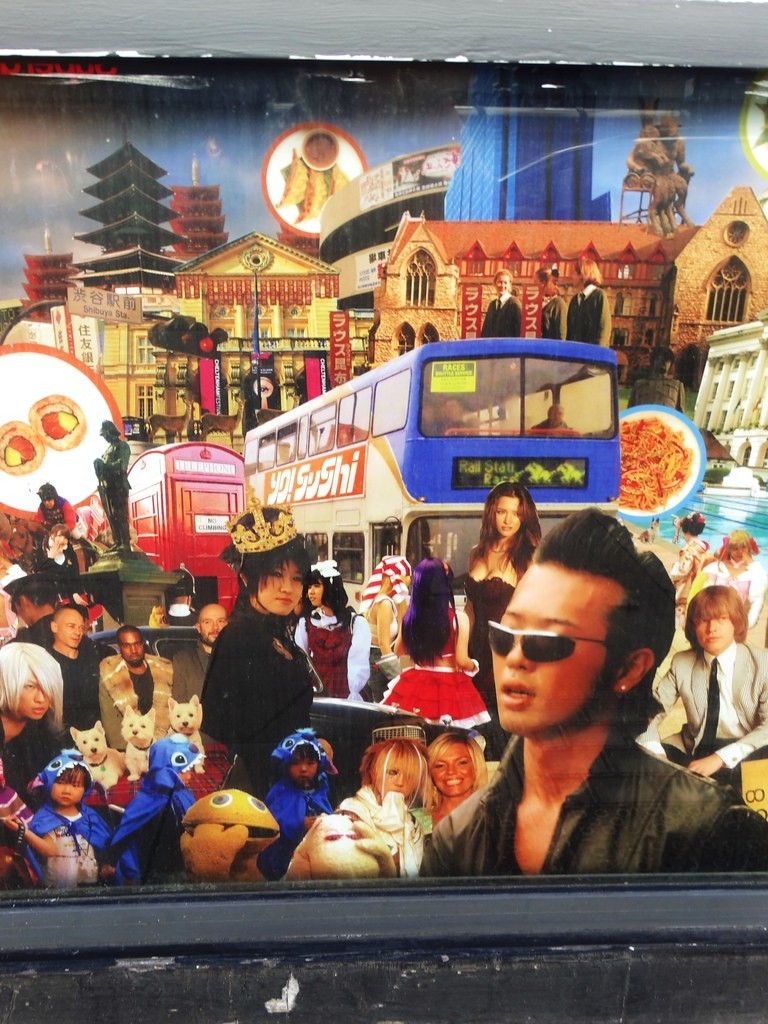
[164,569,195,596]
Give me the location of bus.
[242,337,624,607]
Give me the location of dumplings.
[272,147,350,224]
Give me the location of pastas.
[617,415,695,512]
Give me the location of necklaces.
[63,810,97,878]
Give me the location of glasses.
[486,620,615,662]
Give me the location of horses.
[4,512,109,576]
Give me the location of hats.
[3,573,62,613]
[37,482,59,510]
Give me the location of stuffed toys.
[180,788,281,883]
[285,812,398,880]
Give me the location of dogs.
[69,694,206,790]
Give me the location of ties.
[694,659,720,760]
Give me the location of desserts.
[0,394,86,476]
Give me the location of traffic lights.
[148,314,229,355]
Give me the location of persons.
[530,404,570,438]
[93,420,133,550]
[626,346,685,413]
[481,258,612,347]
[422,393,480,437]
[419,507,768,877]
[635,511,768,792]
[466,481,542,760]
[226,555,492,880]
[199,477,316,800]
[0,480,226,892]
